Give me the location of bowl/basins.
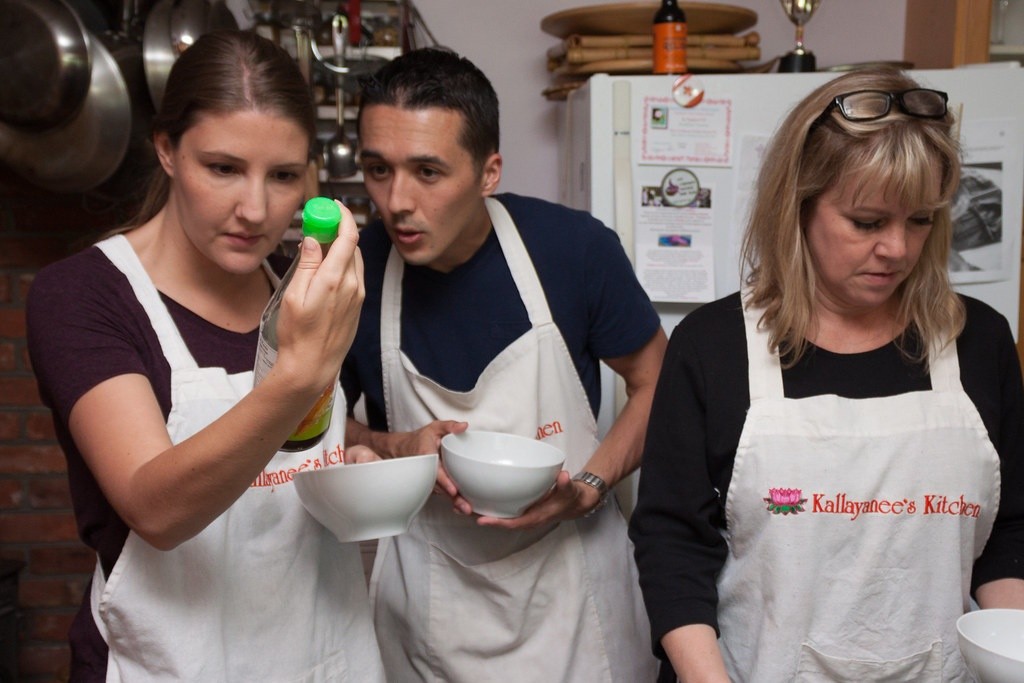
[440,431,565,519]
[955,607,1024,683]
[291,454,439,543]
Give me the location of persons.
[24,33,384,683]
[339,46,669,683]
[628,69,1024,683]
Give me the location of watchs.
[573,472,609,519]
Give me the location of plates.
[821,58,914,72]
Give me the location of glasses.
[805,88,950,148]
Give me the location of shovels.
[322,15,358,178]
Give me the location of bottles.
[253,197,342,452]
[651,0,688,74]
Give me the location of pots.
[1,1,258,204]
